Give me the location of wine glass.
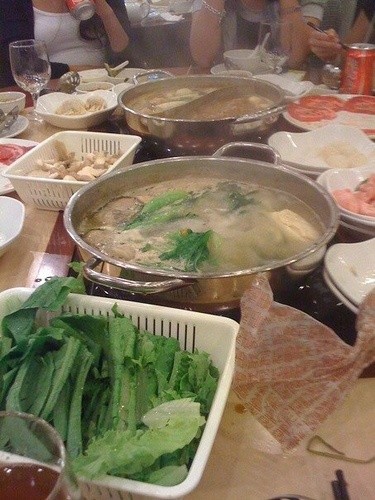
[9,39,51,122]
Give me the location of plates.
[66,67,147,87]
[315,165,375,228]
[284,92,375,140]
[0,139,40,196]
[322,237,375,315]
[210,64,228,75]
[253,74,315,100]
[267,124,375,178]
[0,114,29,138]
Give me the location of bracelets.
[201,0,224,20]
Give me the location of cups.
[215,70,253,78]
[0,408,72,500]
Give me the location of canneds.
[339,43,375,95]
[65,0,96,21]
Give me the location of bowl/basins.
[0,196,25,258]
[111,83,134,96]
[224,49,259,74]
[0,91,26,114]
[73,81,115,94]
[34,91,118,131]
[168,0,195,14]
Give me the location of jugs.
[124,0,151,27]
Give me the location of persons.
[189,0,307,69]
[301,0,375,66]
[0,0,134,87]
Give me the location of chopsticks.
[331,470,348,500]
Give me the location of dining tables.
[0,66,375,500]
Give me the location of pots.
[62,140,340,313]
[118,67,296,156]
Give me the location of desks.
[124,0,195,28]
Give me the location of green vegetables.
[0,262,222,488]
